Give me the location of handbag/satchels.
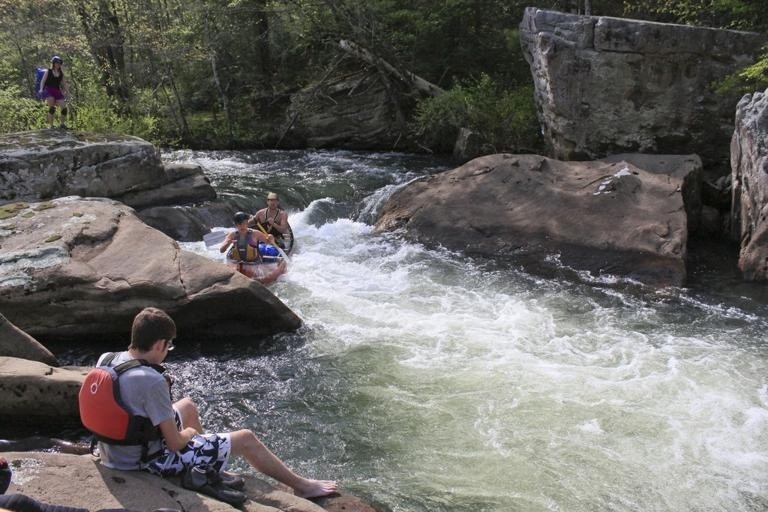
[35,67,47,98]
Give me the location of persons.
[92,304,340,504]
[36,54,70,130]
[247,191,289,251]
[217,210,275,267]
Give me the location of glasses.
[167,342,175,351]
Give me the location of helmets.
[234,211,249,223]
[268,193,279,200]
[53,56,64,64]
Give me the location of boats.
[227,218,294,284]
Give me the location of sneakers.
[181,461,248,505]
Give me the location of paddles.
[253,217,291,264]
[202,222,269,247]
